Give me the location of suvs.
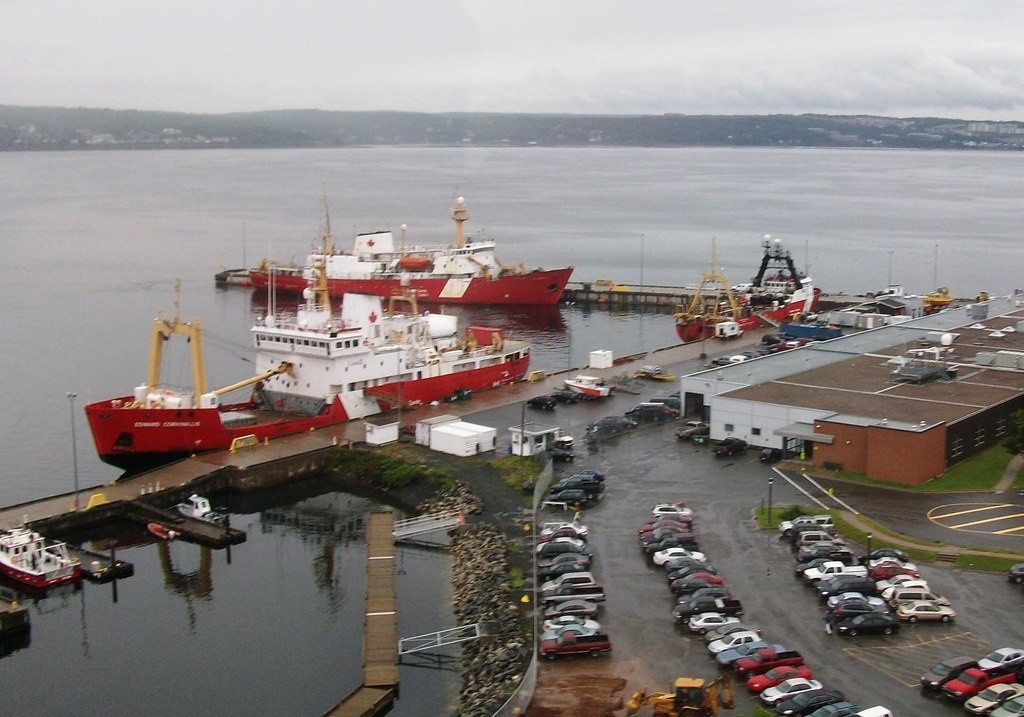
[1008,563,1024,584]
[759,447,783,461]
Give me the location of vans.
[623,402,665,423]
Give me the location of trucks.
[715,322,744,340]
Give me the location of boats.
[147,523,182,540]
[0,513,81,588]
[674,238,821,344]
[178,494,227,525]
[564,375,615,398]
[83,259,530,472]
[250,187,574,306]
[634,365,677,382]
[923,288,954,311]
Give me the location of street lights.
[67,392,80,511]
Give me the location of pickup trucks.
[776,515,833,534]
[538,630,612,661]
[714,436,747,456]
[941,667,1015,703]
[538,584,607,605]
[732,648,805,679]
[675,420,710,438]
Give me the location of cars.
[791,528,958,648]
[638,503,788,665]
[920,646,1024,717]
[587,415,638,436]
[534,523,599,640]
[528,396,555,410]
[547,470,605,504]
[747,665,892,717]
[712,334,816,366]
